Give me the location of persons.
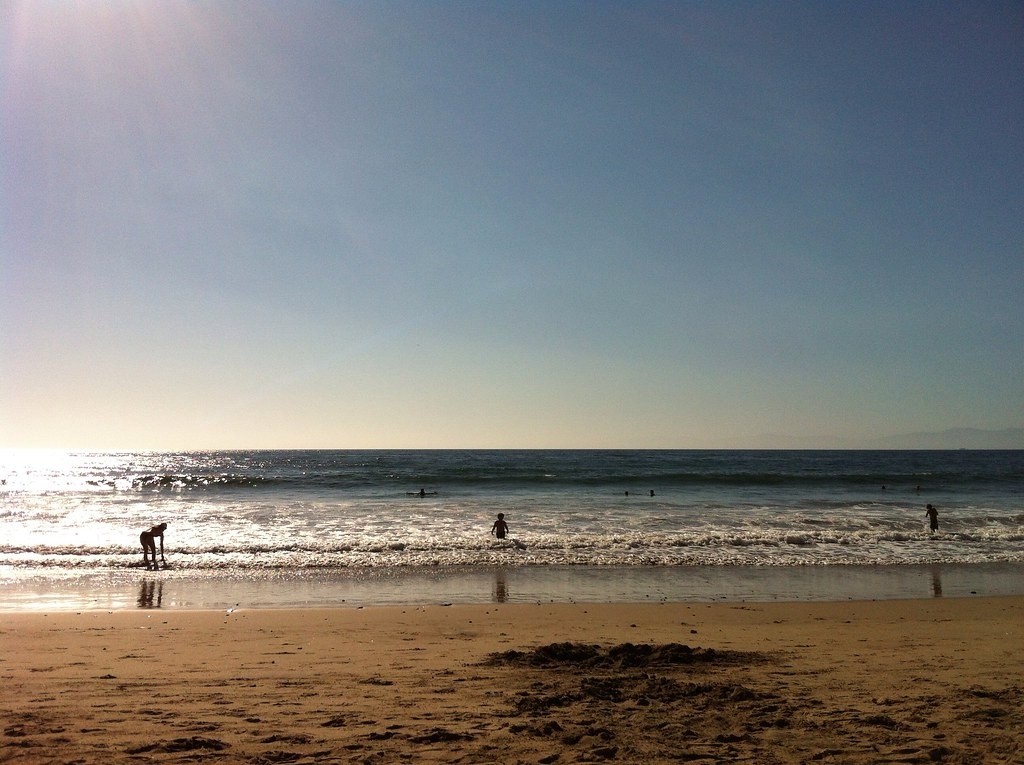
[139,523,168,561]
[925,504,938,533]
[649,489,655,496]
[491,512,509,540]
